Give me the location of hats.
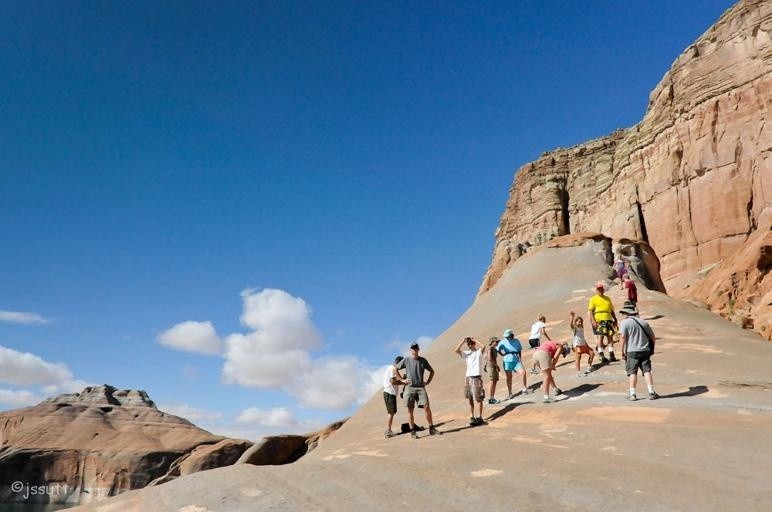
[503,329,514,338]
[619,305,638,315]
[595,283,604,288]
[563,346,570,358]
[489,337,500,345]
[410,341,418,347]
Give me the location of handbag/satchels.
[649,338,654,355]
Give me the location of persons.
[454,336,486,426]
[570,311,595,377]
[497,329,533,400]
[483,336,502,404]
[529,312,552,375]
[393,341,443,439]
[382,356,412,439]
[612,252,628,290]
[588,274,659,400]
[532,340,572,404]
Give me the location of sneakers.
[489,399,500,404]
[648,391,659,400]
[624,394,636,401]
[410,431,418,438]
[429,427,442,435]
[586,367,595,372]
[469,417,483,425]
[599,356,616,363]
[577,373,587,377]
[384,430,399,438]
